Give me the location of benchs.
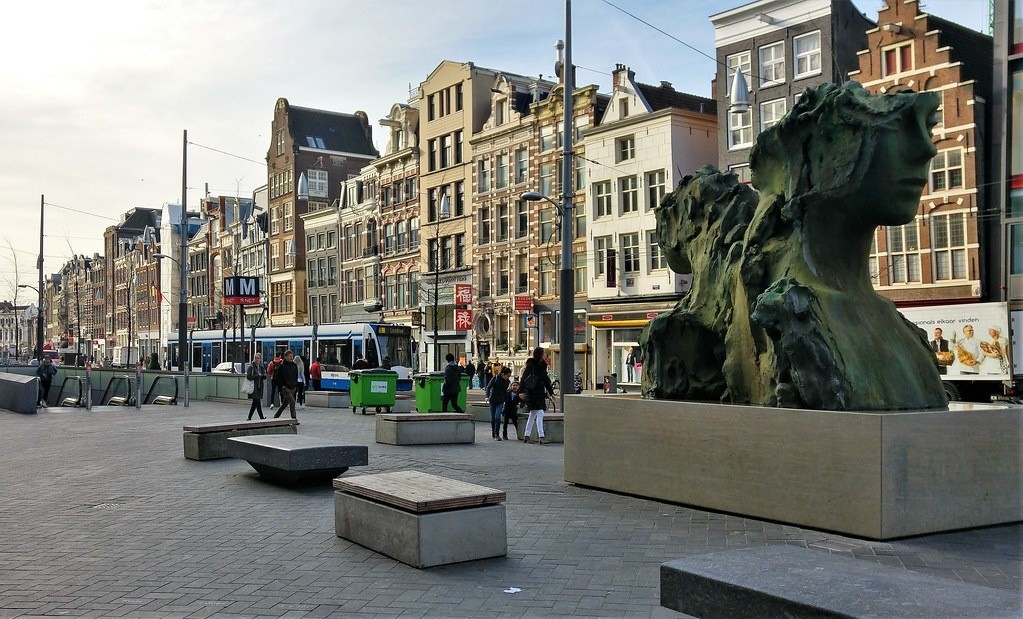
[466,401,526,423]
[226,433,368,490]
[375,412,476,445]
[304,390,349,409]
[183,417,300,462]
[517,413,565,444]
[390,394,411,413]
[333,470,507,570]
[660,545,1023,619]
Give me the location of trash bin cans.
[348,369,399,414]
[412,372,470,413]
[603,373,617,393]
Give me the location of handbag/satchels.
[241,366,254,394]
[524,367,537,388]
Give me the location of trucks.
[894,303,1023,402]
[111,346,138,367]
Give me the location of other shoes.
[295,422,300,425]
[503,436,508,440]
[269,404,274,409]
[495,436,502,441]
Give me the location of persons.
[517,347,556,445]
[267,351,308,409]
[353,353,369,370]
[36,355,58,408]
[310,358,322,391]
[626,347,636,383]
[484,356,530,440]
[465,357,502,389]
[792,92,949,410]
[929,328,956,375]
[140,352,161,370]
[273,350,300,425]
[247,353,267,421]
[381,356,391,370]
[441,353,464,413]
[951,324,985,373]
[982,325,1010,374]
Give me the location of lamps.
[728,67,752,114]
[37,254,40,269]
[439,192,450,220]
[297,171,309,201]
[288,237,296,257]
[142,225,151,246]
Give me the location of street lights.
[18,284,43,360]
[520,192,576,414]
[152,254,188,371]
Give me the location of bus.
[166,290,413,392]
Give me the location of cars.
[43,349,60,365]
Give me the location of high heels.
[539,437,550,444]
[523,436,535,444]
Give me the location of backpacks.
[272,360,283,380]
